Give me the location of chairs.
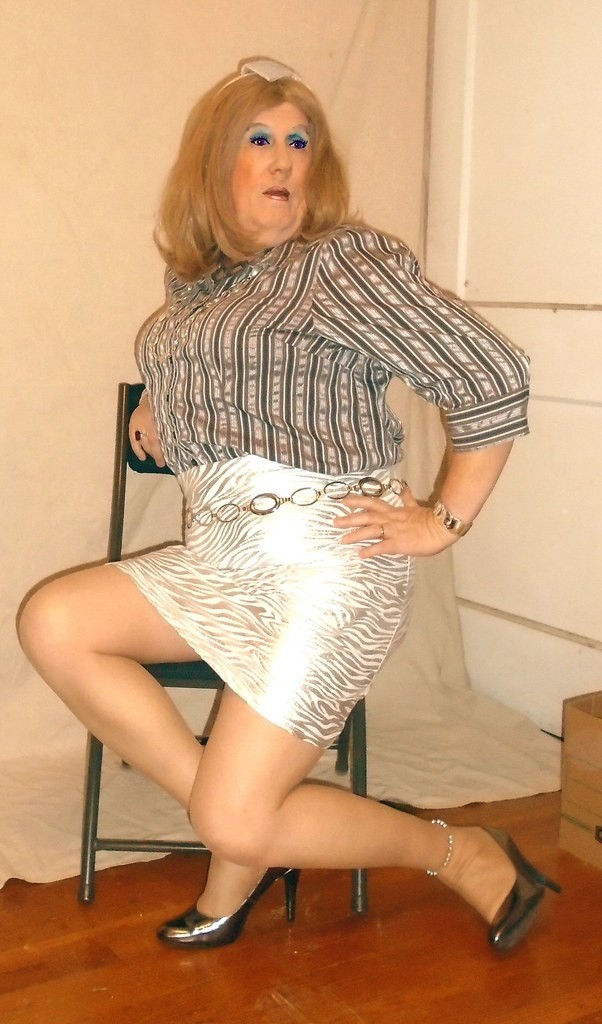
[78,381,370,912]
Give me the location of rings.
[380,524,385,538]
[135,431,145,440]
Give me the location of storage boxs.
[558,692,602,869]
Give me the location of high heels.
[157,861,301,944]
[470,824,562,948]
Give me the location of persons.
[21,55,565,949]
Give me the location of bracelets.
[426,492,473,536]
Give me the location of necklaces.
[149,261,266,368]
